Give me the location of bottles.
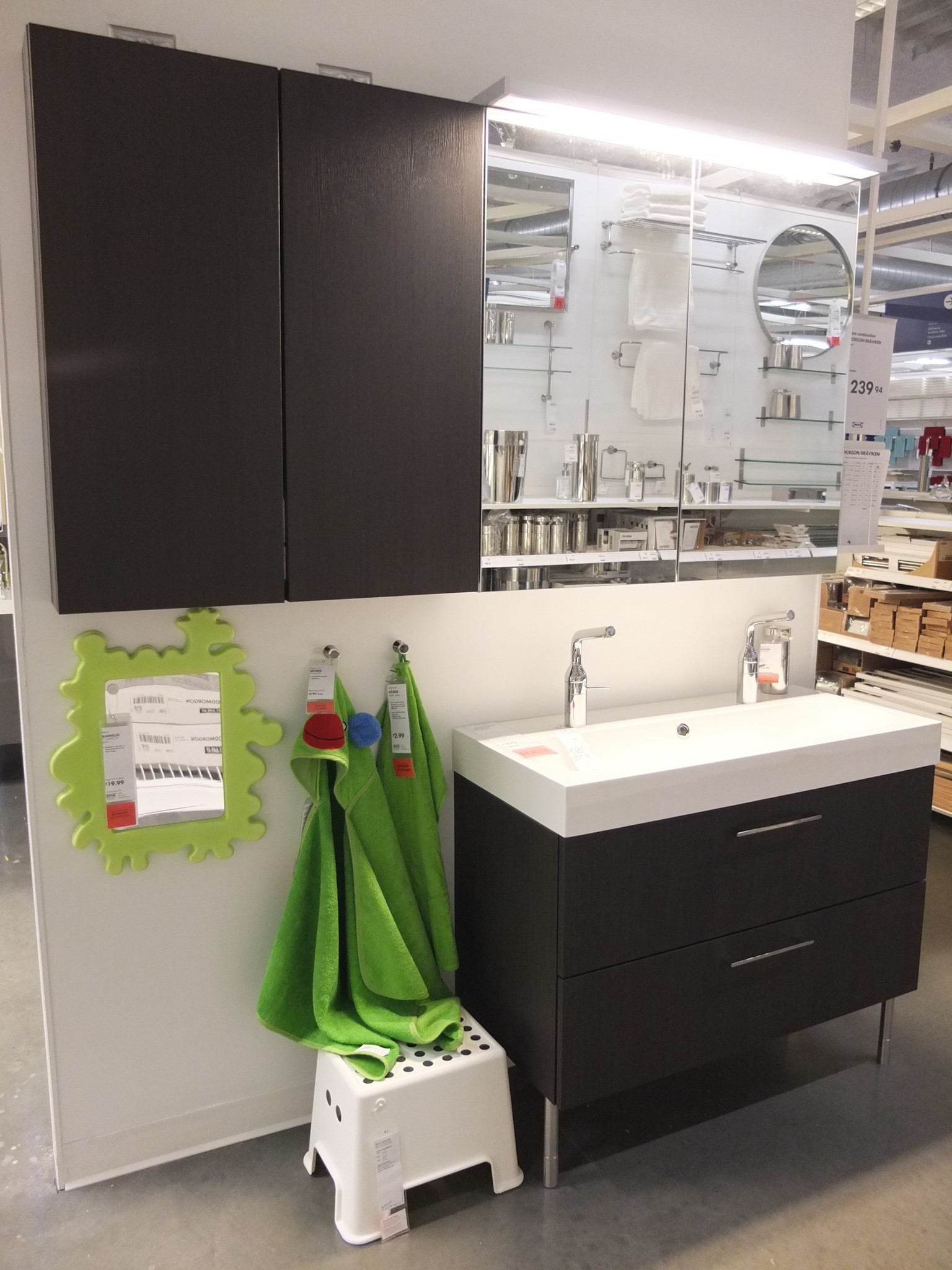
[483,429,599,503]
[481,509,592,557]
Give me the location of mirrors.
[478,117,862,592]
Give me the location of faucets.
[735,610,795,705]
[562,626,619,728]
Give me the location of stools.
[301,1006,525,1245]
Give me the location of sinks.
[446,684,942,837]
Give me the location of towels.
[256,666,465,1082]
[621,181,707,208]
[622,202,707,229]
[630,339,704,420]
[362,653,463,974]
[628,248,695,334]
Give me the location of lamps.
[490,94,879,180]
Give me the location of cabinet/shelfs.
[443,763,943,1194]
[22,20,482,615]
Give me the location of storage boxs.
[815,538,952,820]
